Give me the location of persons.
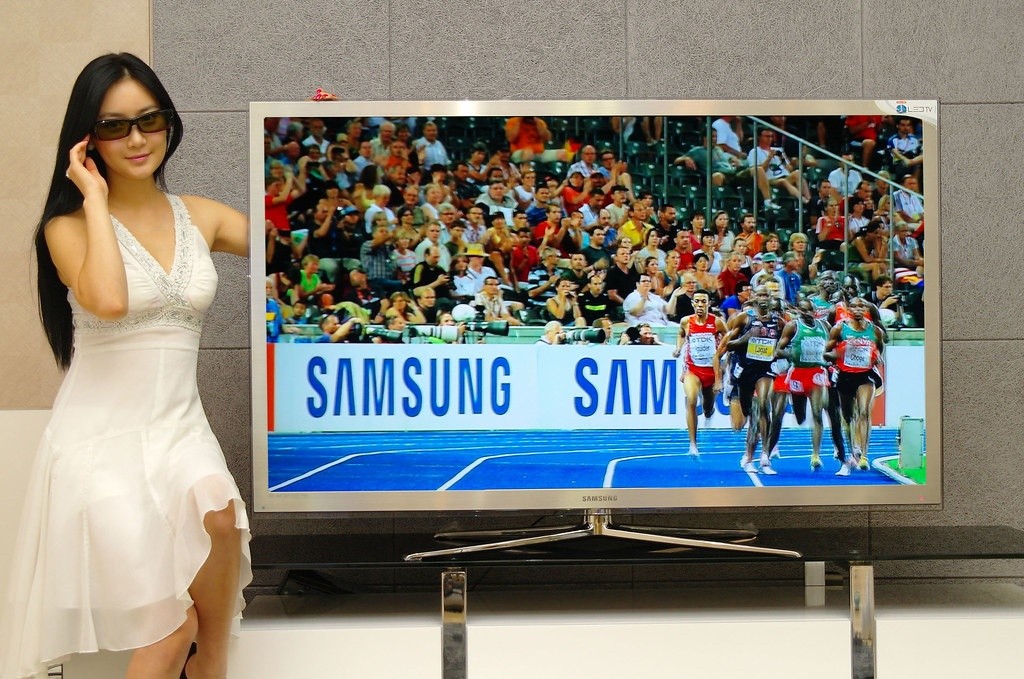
[263,115,924,347]
[673,269,889,476]
[0,52,340,679]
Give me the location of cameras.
[565,292,574,300]
[559,328,606,345]
[460,320,510,336]
[403,323,458,341]
[352,321,403,344]
[775,149,782,157]
[893,293,904,306]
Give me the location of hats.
[762,251,778,263]
[752,252,763,264]
[701,227,714,236]
[610,185,628,192]
[458,243,492,257]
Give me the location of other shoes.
[859,457,869,471]
[771,447,780,460]
[833,449,839,458]
[854,447,862,460]
[760,453,770,465]
[741,456,757,473]
[835,464,851,477]
[810,453,821,468]
[765,203,781,211]
[689,443,700,457]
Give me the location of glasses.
[90,108,176,141]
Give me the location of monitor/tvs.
[249,99,943,561]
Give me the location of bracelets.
[571,302,579,305]
